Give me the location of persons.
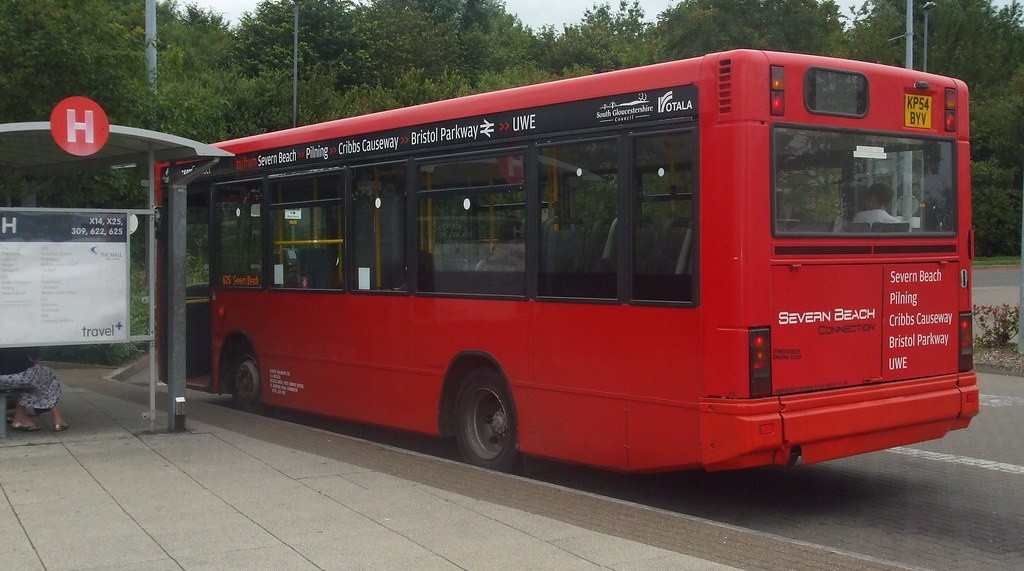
[0,347,70,431]
[485,215,525,272]
[252,221,279,257]
[851,183,902,223]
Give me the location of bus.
[154,47,980,477]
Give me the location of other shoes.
[7,410,39,423]
[12,423,42,431]
[54,422,71,432]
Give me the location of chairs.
[871,223,910,232]
[648,218,690,299]
[844,222,871,233]
[585,217,612,276]
[582,216,658,299]
[788,219,832,232]
[675,220,695,301]
[537,218,586,296]
